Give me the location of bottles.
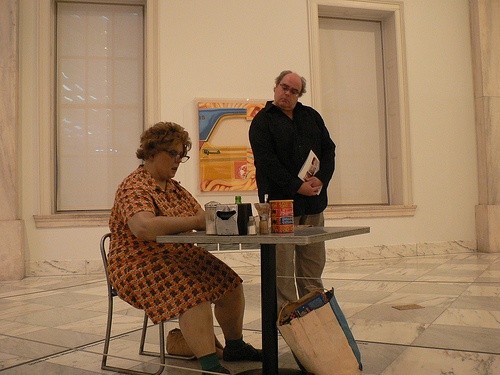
[247,216,257,236]
[235,196,241,204]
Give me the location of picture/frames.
[193,96,270,195]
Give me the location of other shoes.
[201,364,231,375]
[222,342,262,362]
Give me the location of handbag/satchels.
[276,283,363,375]
[166,328,225,357]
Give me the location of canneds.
[254,202,271,234]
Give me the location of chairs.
[99,233,208,375]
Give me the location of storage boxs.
[215,203,253,236]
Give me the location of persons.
[248,71,337,313]
[107,122,263,375]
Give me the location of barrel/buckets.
[204,201,271,236]
[269,200,294,233]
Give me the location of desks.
[157,222,370,374]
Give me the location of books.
[298,149,323,195]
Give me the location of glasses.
[159,148,190,163]
[281,86,301,97]
